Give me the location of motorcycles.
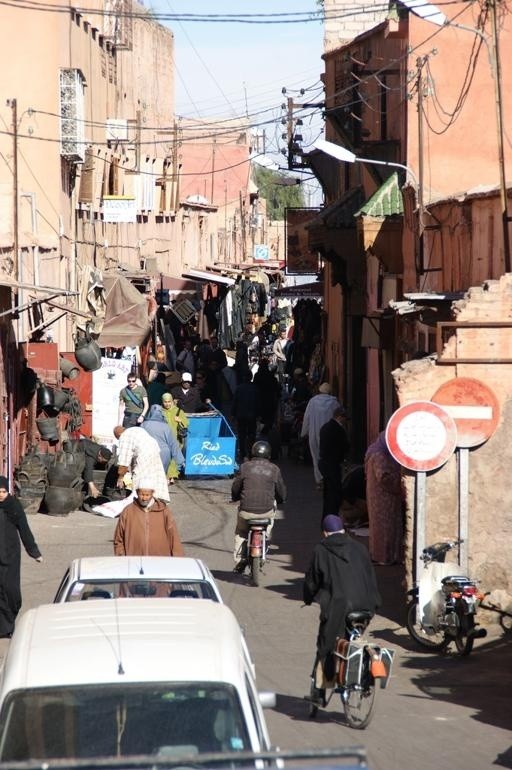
[284,398,316,469]
[406,533,491,656]
[231,489,280,587]
[247,303,293,368]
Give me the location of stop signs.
[429,381,504,457]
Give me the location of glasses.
[128,379,136,383]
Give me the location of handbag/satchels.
[175,418,190,438]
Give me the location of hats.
[100,446,113,461]
[0,474,10,492]
[113,425,126,440]
[180,371,195,383]
[160,392,174,401]
[134,474,158,493]
[321,513,345,534]
[317,382,333,395]
[294,368,304,375]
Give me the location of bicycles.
[299,594,386,733]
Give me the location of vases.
[12,338,134,518]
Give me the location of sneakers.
[233,557,250,575]
[303,690,326,710]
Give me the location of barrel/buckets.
[13,440,108,517]
[71,332,103,371]
[36,382,84,443]
[59,358,81,381]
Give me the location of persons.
[0,475,43,638]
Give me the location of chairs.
[168,588,199,600]
[79,590,112,600]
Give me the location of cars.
[0,587,282,767]
[48,552,225,633]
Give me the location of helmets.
[251,440,274,459]
